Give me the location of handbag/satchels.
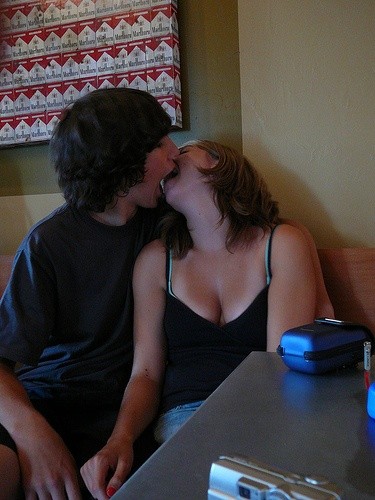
[277,318,375,372]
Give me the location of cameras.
[207,452,345,500]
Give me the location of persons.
[80,138,317,500]
[0,88,335,500]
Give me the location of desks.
[109,350,374,500]
